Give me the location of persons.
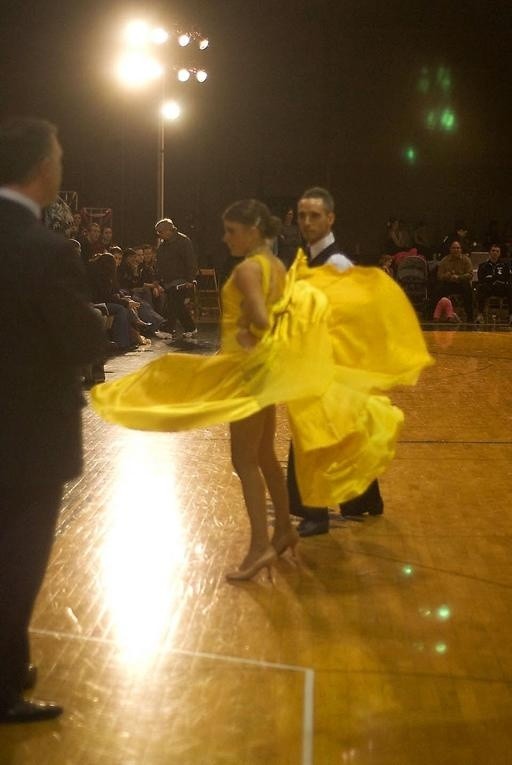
[0,117,109,723]
[274,207,299,268]
[66,212,199,353]
[286,188,384,538]
[384,217,512,325]
[88,200,438,585]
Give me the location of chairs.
[384,249,511,332]
[187,268,222,319]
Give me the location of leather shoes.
[297,521,328,535]
[0,697,62,721]
[340,496,382,515]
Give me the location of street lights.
[119,13,209,243]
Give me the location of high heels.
[227,529,297,583]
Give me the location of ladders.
[193,268,221,324]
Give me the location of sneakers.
[182,329,198,337]
[155,331,172,339]
